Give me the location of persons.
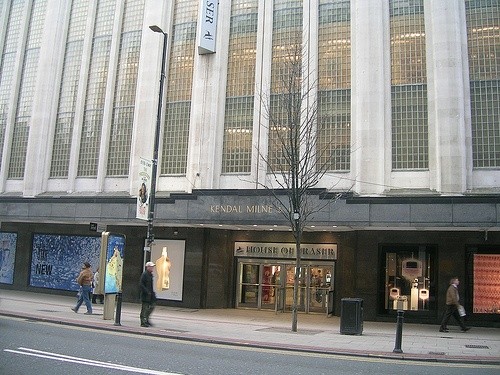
[70,262,95,315]
[92,267,104,304]
[139,262,156,327]
[439,278,468,333]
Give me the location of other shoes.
[462,328,471,332]
[141,322,151,327]
[92,301,97,304]
[72,307,78,312]
[439,328,449,332]
[85,311,91,315]
[100,301,104,304]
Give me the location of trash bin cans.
[340,297,363,336]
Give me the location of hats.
[84,262,91,268]
[145,262,156,267]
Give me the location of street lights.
[144,24,168,263]
